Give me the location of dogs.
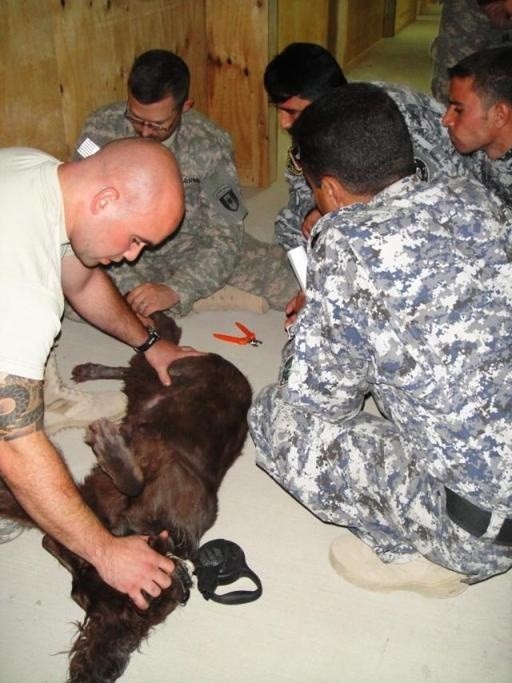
[0,290,253,683]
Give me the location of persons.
[429,0,512,104]
[263,41,485,253]
[441,45,512,209]
[245,80,512,598]
[1,136,210,612]
[72,49,303,317]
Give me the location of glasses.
[122,111,175,132]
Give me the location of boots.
[36,347,130,437]
[191,285,270,313]
[329,533,469,597]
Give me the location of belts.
[445,489,510,544]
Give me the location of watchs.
[133,325,162,353]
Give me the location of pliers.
[212,320,262,348]
